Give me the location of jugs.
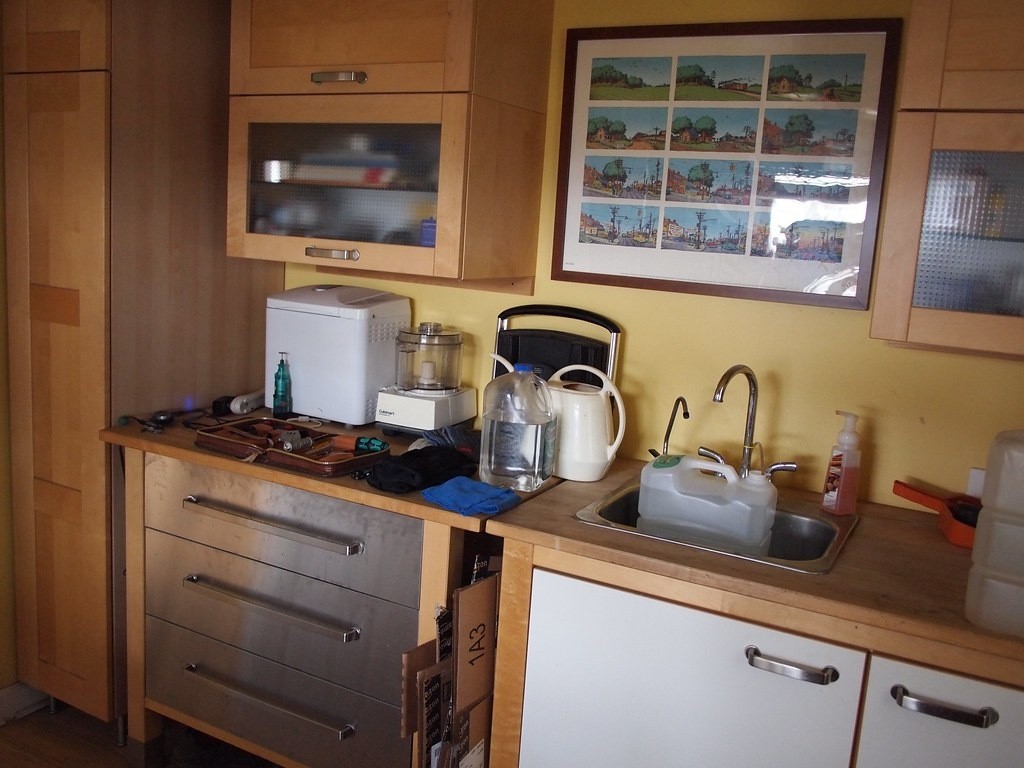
[488,353,626,482]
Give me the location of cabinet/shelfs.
[226,0,554,295]
[97,394,1024,768]
[868,0,1024,357]
[0,0,283,727]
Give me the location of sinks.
[575,465,862,578]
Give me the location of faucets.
[662,395,690,454]
[712,364,760,480]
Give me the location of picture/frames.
[551,15,905,312]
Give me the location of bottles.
[965,430,1024,637]
[636,454,777,555]
[479,364,558,493]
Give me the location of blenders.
[375,321,478,443]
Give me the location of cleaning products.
[818,409,862,516]
